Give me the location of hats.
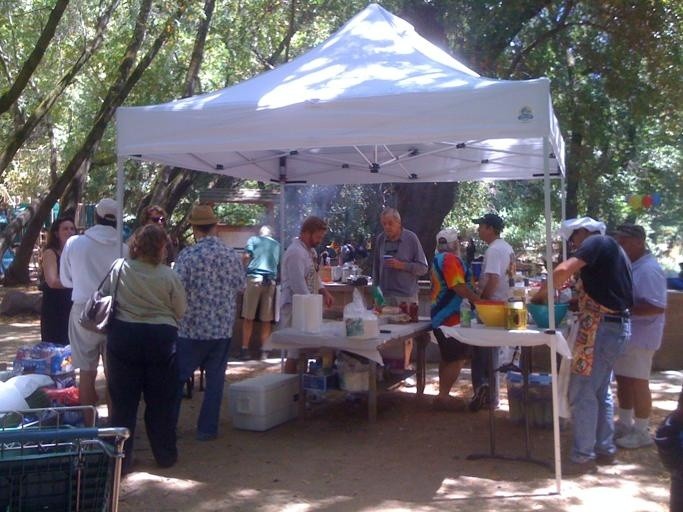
[613,224,645,237]
[436,230,456,243]
[559,217,606,241]
[472,214,502,226]
[190,205,218,224]
[95,198,116,221]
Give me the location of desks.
[432,321,572,510]
[265,320,439,424]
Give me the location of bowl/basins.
[472,299,509,329]
[526,302,570,330]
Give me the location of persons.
[238,224,283,361]
[371,209,428,388]
[142,204,167,226]
[106,222,186,470]
[39,216,75,347]
[607,222,669,449]
[171,204,248,442]
[535,217,636,476]
[430,213,521,411]
[59,198,130,428]
[279,216,333,374]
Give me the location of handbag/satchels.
[78,291,115,333]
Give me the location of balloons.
[651,192,661,205]
[641,195,653,207]
[628,193,644,211]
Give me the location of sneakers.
[614,422,653,448]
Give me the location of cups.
[382,254,394,272]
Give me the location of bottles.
[0,363,8,382]
[10,341,73,378]
[458,298,472,329]
[309,361,316,372]
[399,301,418,324]
[322,262,330,282]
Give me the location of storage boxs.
[339,369,376,392]
[226,371,303,433]
[380,339,412,374]
[505,371,554,429]
[301,373,338,393]
